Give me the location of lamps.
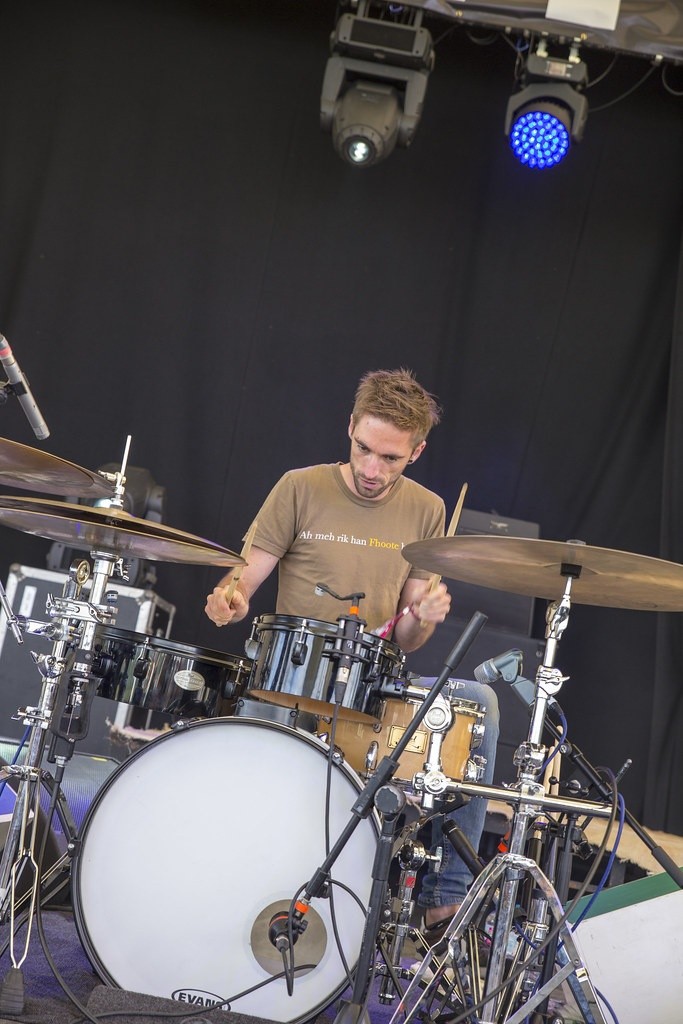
[504,39,593,171]
[320,0,436,167]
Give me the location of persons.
[204,365,500,960]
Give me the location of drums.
[91,618,254,718]
[67,713,392,1024]
[245,610,410,728]
[314,682,489,801]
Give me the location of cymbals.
[0,430,119,502]
[0,488,251,571]
[400,530,683,614]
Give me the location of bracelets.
[412,610,421,622]
